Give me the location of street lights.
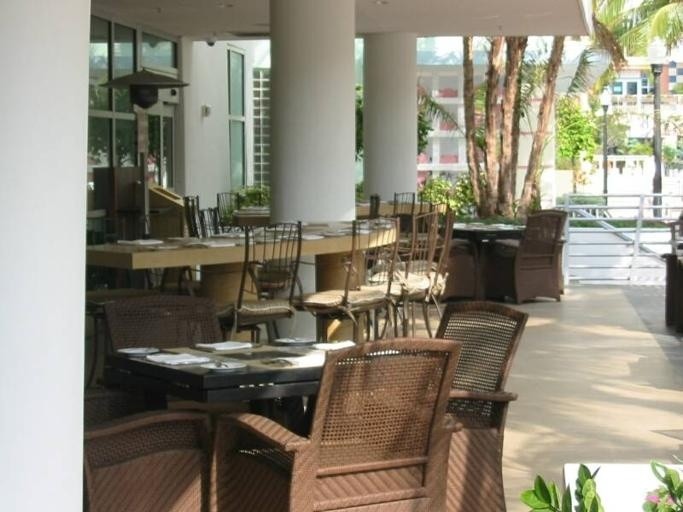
[599,86,613,194]
[647,34,666,218]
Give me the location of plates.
[115,347,159,357]
[167,234,212,248]
[273,337,316,345]
[200,361,247,374]
[215,216,395,246]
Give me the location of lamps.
[97,68,190,110]
